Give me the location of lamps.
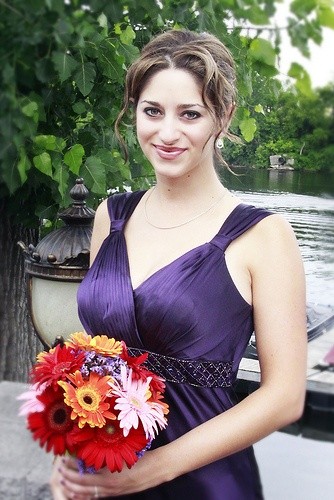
[17,174,100,349]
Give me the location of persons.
[48,28,308,500]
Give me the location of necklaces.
[144,185,229,230]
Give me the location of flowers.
[16,331,169,477]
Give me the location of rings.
[94,485,99,499]
